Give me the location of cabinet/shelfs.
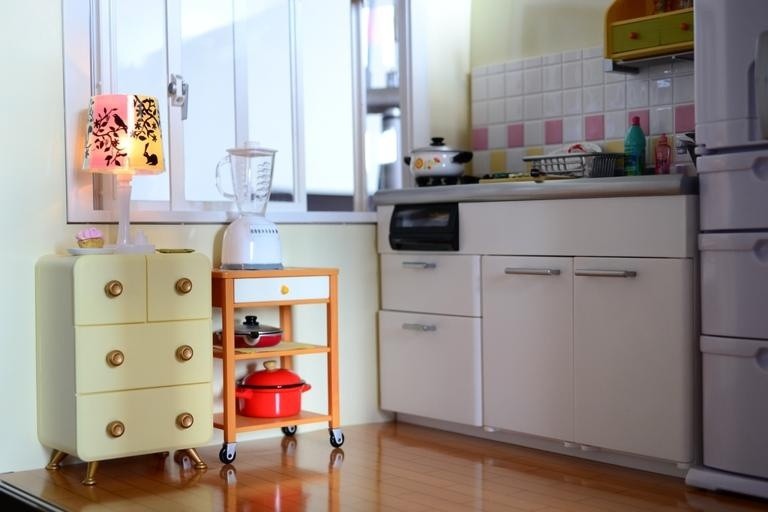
[212,268,345,464]
[480,255,701,465]
[378,253,484,427]
[36,252,215,488]
[602,0,694,59]
[695,146,768,482]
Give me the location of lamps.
[81,92,165,252]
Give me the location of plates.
[537,140,604,178]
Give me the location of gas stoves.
[416,176,479,188]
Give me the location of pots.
[403,133,474,183]
[217,312,285,351]
[232,359,314,421]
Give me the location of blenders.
[212,144,290,270]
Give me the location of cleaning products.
[623,117,672,175]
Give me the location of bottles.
[619,118,648,178]
[652,130,673,174]
